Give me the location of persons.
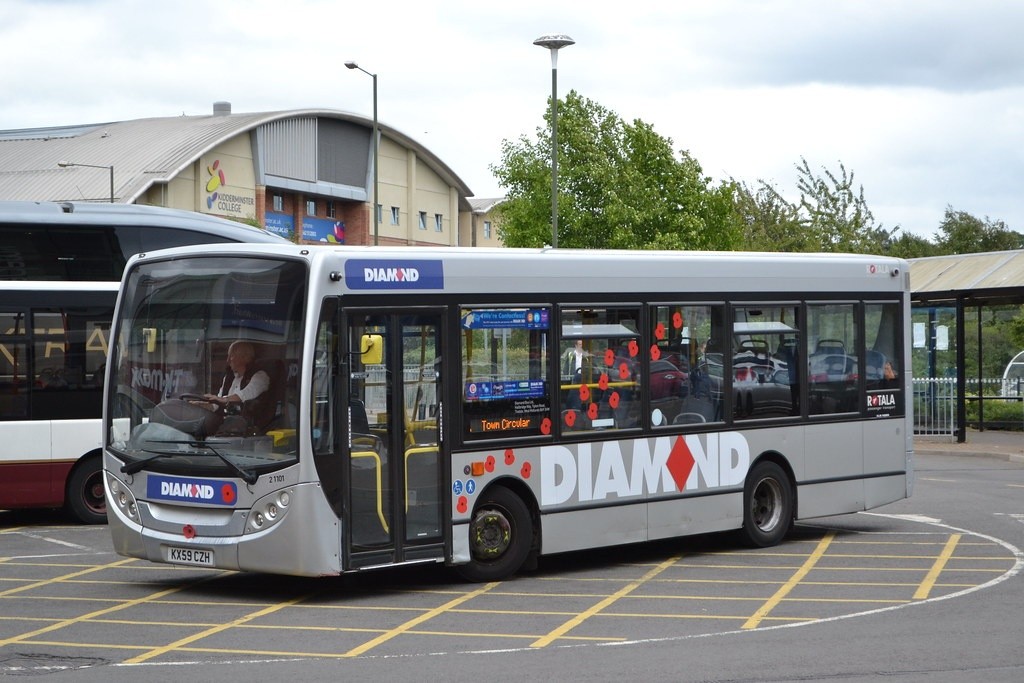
[563,340,582,374]
[189,340,276,436]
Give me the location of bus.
[94,245,917,583]
[0,204,337,528]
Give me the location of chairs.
[567,336,885,399]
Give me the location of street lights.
[57,162,121,205]
[346,62,382,242]
[533,33,578,244]
